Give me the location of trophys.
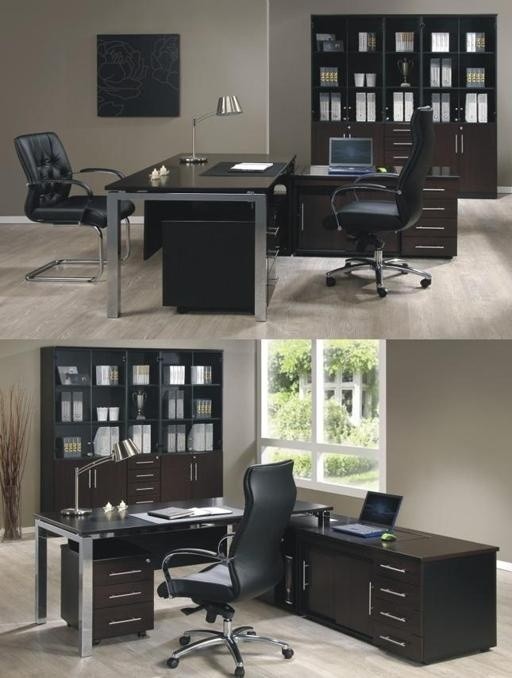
[132,388,147,419]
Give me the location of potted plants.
[0,380,33,541]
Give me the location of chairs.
[15,133,133,284]
[323,105,434,298]
[158,460,297,677]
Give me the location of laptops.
[328,137,374,175]
[331,490,404,538]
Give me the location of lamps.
[180,95,244,163]
[60,437,141,516]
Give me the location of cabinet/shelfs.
[432,124,497,199]
[127,459,158,506]
[312,123,382,173]
[42,348,221,453]
[254,525,302,612]
[53,462,127,513]
[59,540,154,641]
[294,179,401,257]
[401,172,457,257]
[160,454,222,502]
[311,12,498,125]
[263,179,294,256]
[162,208,280,316]
[384,124,412,169]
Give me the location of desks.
[103,151,298,321]
[32,496,332,656]
[276,515,497,666]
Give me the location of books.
[191,366,212,383]
[164,424,186,452]
[56,437,81,459]
[396,32,414,52]
[94,426,119,457]
[466,68,486,88]
[148,506,194,519]
[96,365,119,385]
[193,399,211,418]
[187,507,233,517]
[356,93,376,121]
[465,93,488,122]
[467,32,485,52]
[164,365,185,385]
[128,425,151,454]
[393,92,413,122]
[188,423,214,452]
[163,389,184,419]
[432,32,449,52]
[319,92,341,121]
[431,57,451,87]
[359,32,376,51]
[431,93,450,122]
[61,392,83,422]
[131,365,150,385]
[320,67,337,87]
[230,162,274,172]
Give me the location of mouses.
[376,167,388,173]
[381,533,397,542]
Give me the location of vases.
[0,486,23,541]
